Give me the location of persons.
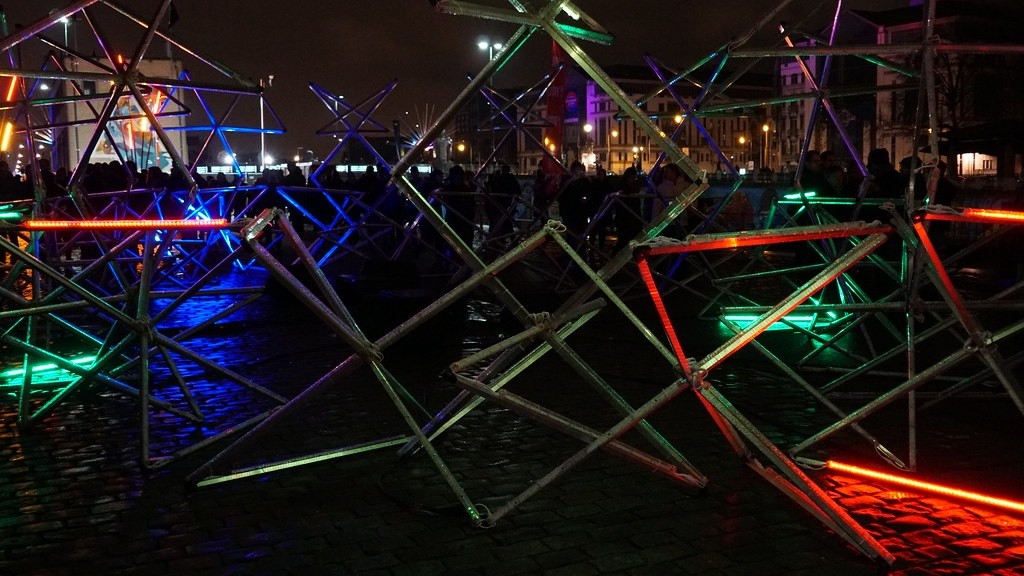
[1,147,1024,332]
